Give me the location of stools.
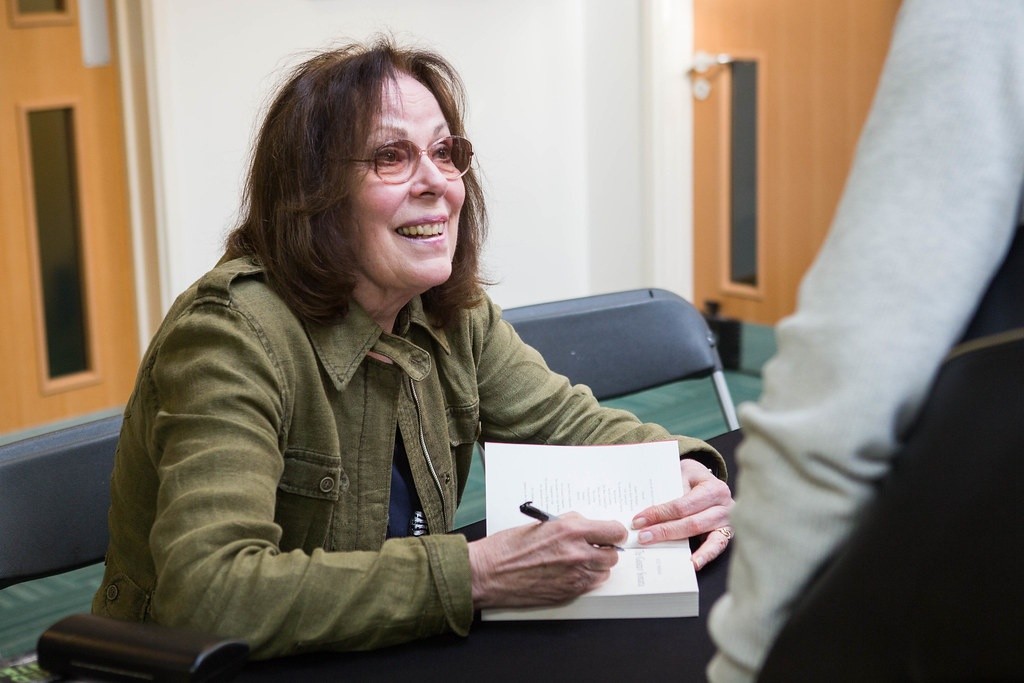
[2,414,132,589]
[501,288,742,440]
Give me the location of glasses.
[322,136,474,185]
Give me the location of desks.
[0,432,745,683]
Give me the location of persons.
[91,39,737,662]
[705,0,1024,683]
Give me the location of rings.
[716,527,731,540]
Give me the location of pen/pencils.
[519,502,626,553]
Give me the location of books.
[481,440,700,619]
